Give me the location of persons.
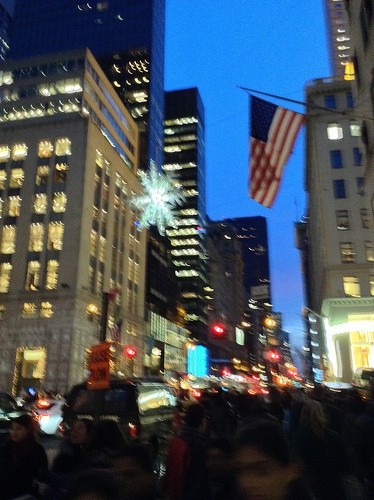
[108,448,161,500]
[0,415,49,500]
[165,381,373,499]
[49,418,95,500]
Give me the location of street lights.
[301,305,328,386]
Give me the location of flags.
[247,96,305,211]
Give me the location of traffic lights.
[243,318,255,328]
[264,316,279,328]
[125,346,137,358]
[213,324,225,336]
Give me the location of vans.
[56,381,189,486]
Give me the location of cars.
[25,389,66,440]
[0,392,36,452]
